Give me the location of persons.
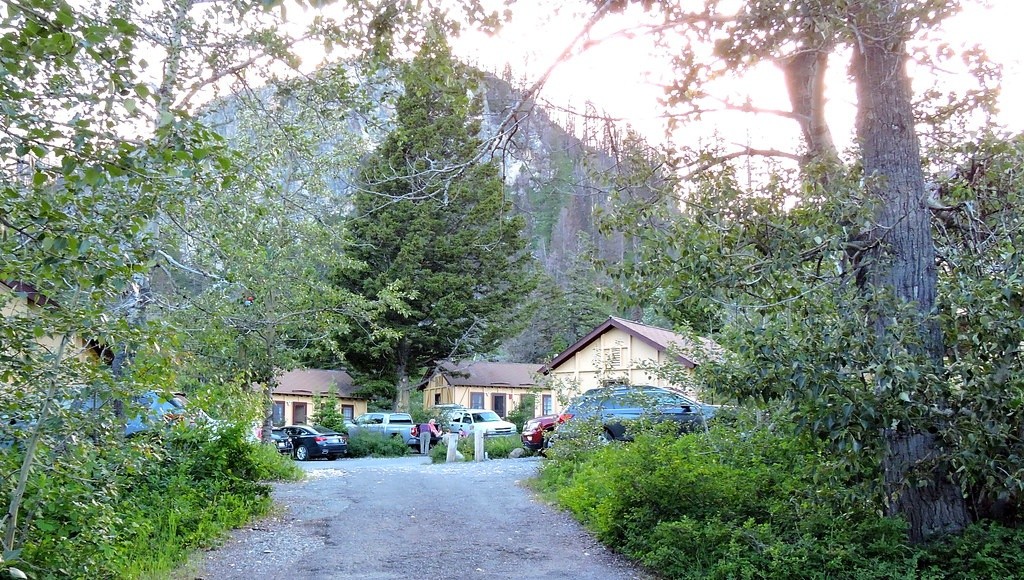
[428,419,442,437]
[418,423,431,456]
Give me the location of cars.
[520,414,561,450]
[253,427,294,452]
[555,384,753,446]
[0,384,254,492]
[431,402,517,455]
[279,423,349,462]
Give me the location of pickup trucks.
[342,412,444,452]
